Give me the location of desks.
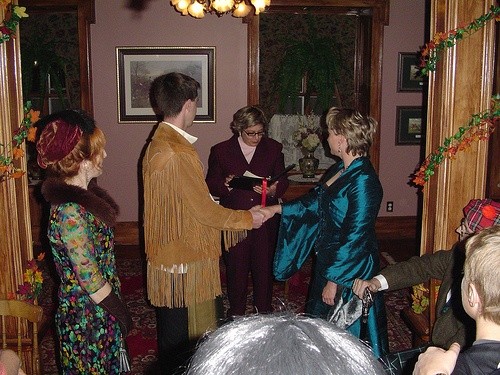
[287,174,323,184]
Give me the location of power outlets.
[387,202,393,212]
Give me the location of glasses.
[461,218,473,237]
[241,127,266,136]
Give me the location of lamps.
[171,0,271,19]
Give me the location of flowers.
[411,282,441,314]
[413,93,500,187]
[418,5,500,76]
[7,252,49,305]
[0,101,41,183]
[0,0,29,44]
[292,110,323,153]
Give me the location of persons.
[351,198,500,375]
[450,226,500,374]
[36,109,132,375]
[182,312,393,375]
[142,72,265,375]
[413,342,461,374]
[251,106,390,360]
[205,104,289,318]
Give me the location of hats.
[462,198,500,231]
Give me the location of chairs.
[0,300,44,375]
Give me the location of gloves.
[96,286,134,336]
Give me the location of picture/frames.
[299,152,319,178]
[397,51,429,93]
[395,106,424,146]
[116,45,217,124]
